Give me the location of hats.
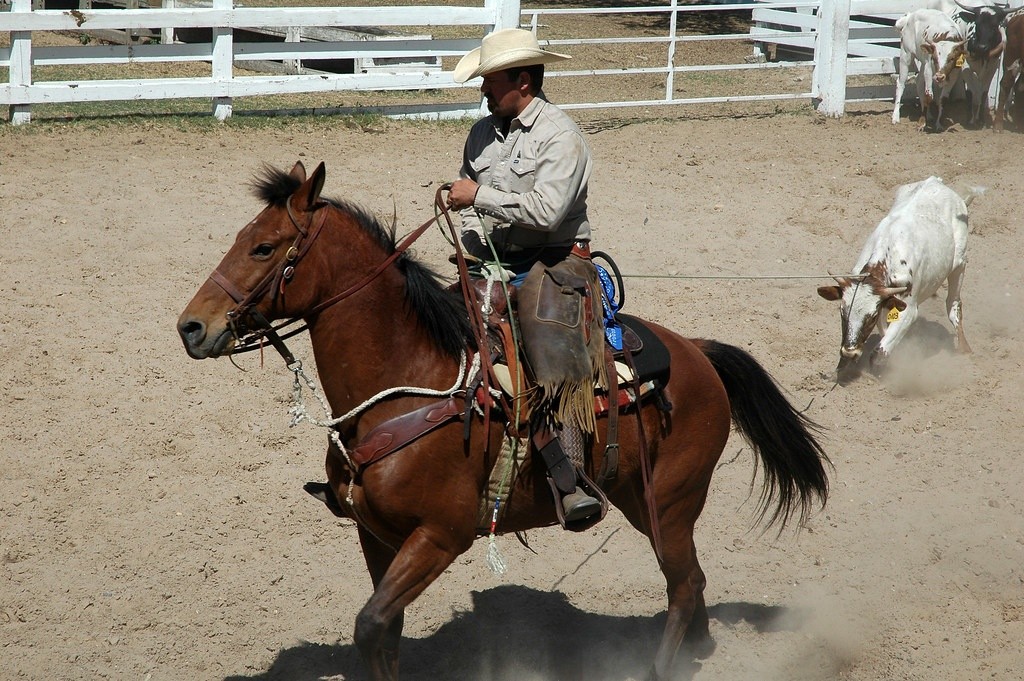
[453,29,572,83]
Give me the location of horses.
[177,160,832,681]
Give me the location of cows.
[890,0,1024,135]
[817,174,975,375]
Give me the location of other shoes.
[562,485,602,521]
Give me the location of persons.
[448,29,605,531]
